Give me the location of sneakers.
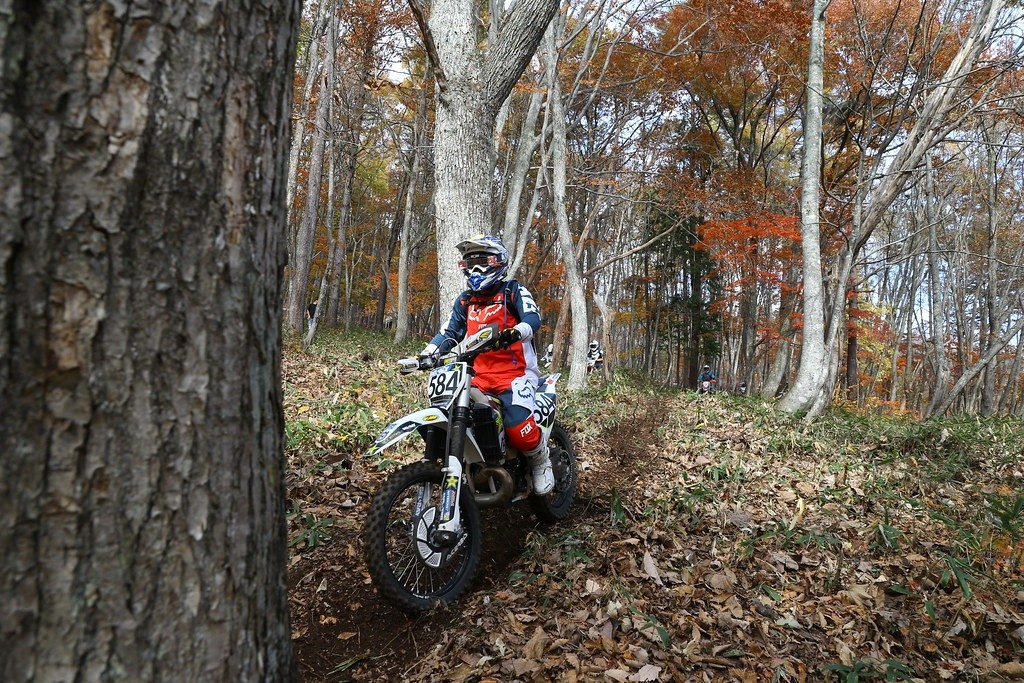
[522,426,556,496]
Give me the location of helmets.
[455,235,508,291]
[589,341,599,351]
[547,344,554,356]
[704,364,710,374]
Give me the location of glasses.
[458,258,500,268]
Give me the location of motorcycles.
[355,323,581,608]
[700,379,715,393]
[587,357,603,370]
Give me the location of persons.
[698,365,716,389]
[587,340,603,367]
[413,235,555,495]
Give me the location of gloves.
[497,328,521,350]
